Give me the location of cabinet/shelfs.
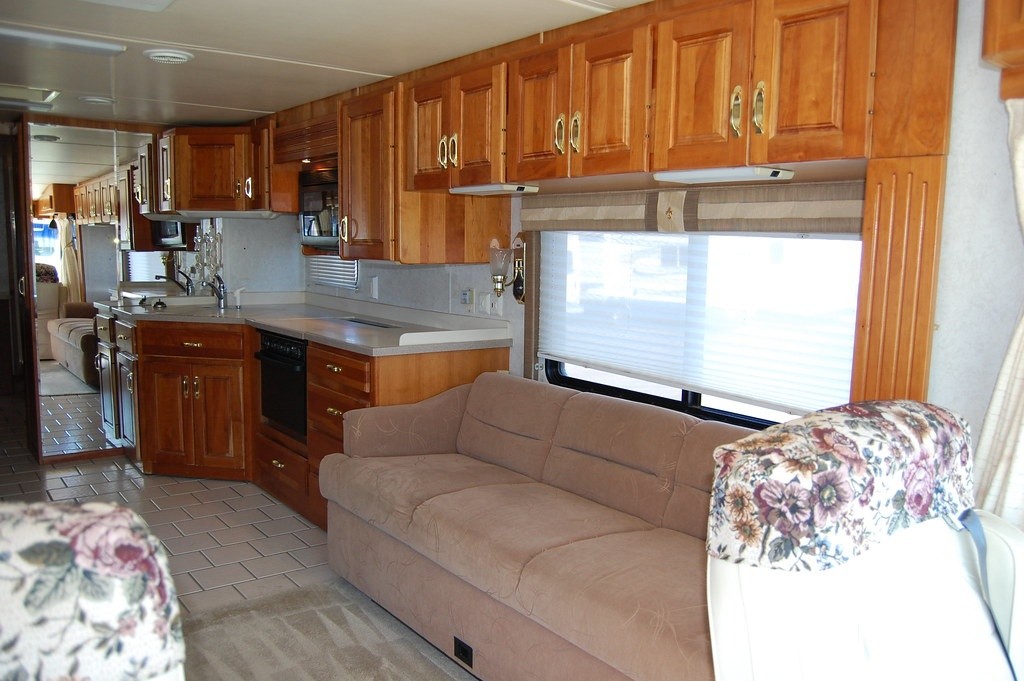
[34,136,153,253]
[115,315,143,474]
[159,125,299,215]
[305,341,509,535]
[252,420,308,520]
[136,326,248,483]
[337,0,879,263]
[95,308,123,448]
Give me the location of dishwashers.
[252,329,307,436]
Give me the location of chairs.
[705,398,1024,681]
[35,263,59,359]
[0,498,186,681]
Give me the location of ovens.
[149,219,188,249]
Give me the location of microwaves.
[296,167,339,251]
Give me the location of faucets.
[154,270,195,296]
[201,273,228,309]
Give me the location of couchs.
[318,372,760,681]
[47,300,100,388]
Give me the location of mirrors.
[23,122,212,458]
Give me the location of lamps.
[489,238,527,304]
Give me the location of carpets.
[181,573,480,681]
[38,358,101,396]
